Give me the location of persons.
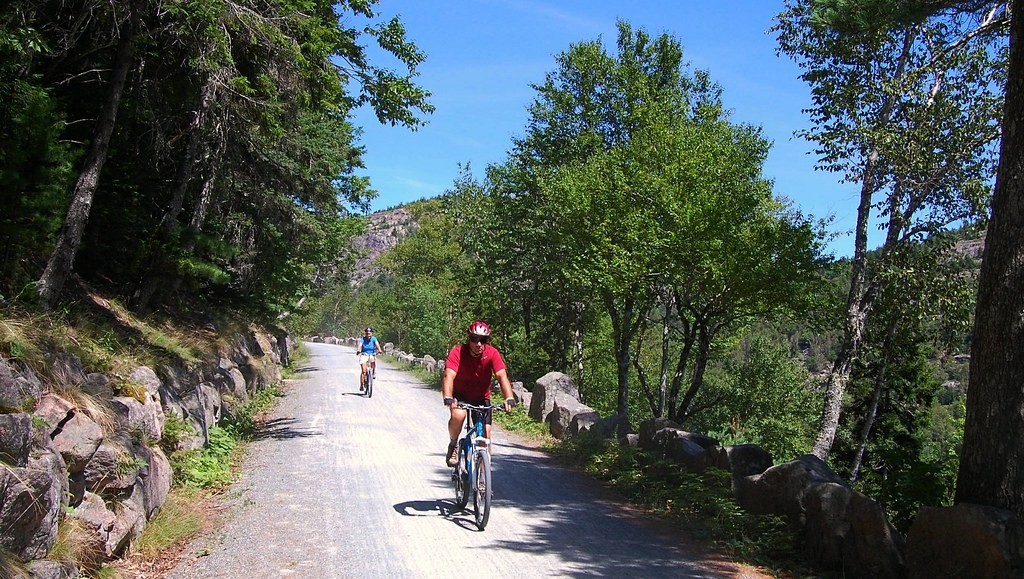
[442,321,515,495]
[357,328,382,391]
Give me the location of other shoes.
[372,373,376,379]
[360,384,365,391]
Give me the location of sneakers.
[479,482,492,499]
[446,443,459,466]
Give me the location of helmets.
[468,321,490,337]
[365,328,373,332]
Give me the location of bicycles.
[359,351,381,398]
[446,397,511,531]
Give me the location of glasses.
[469,336,488,345]
[367,333,371,335]
